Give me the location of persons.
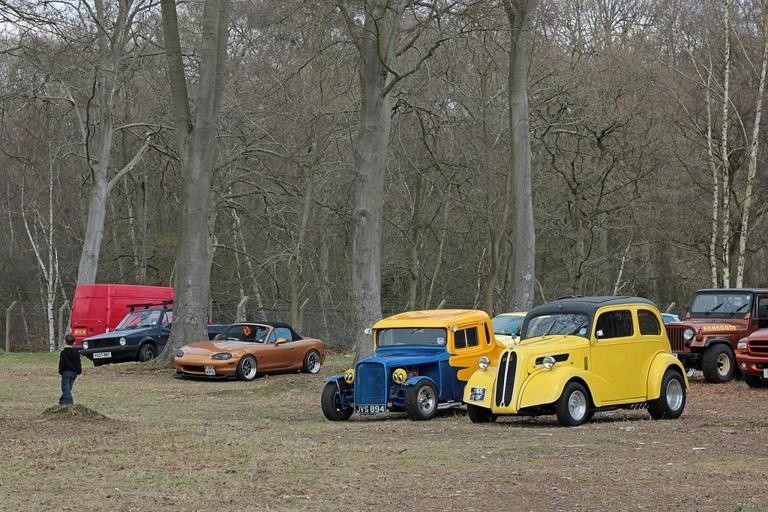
[238,325,256,341]
[57,334,82,405]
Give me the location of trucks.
[69,284,213,353]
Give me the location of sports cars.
[174,322,326,381]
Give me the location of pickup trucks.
[79,298,231,367]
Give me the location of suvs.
[663,287,768,383]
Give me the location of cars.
[321,295,689,425]
